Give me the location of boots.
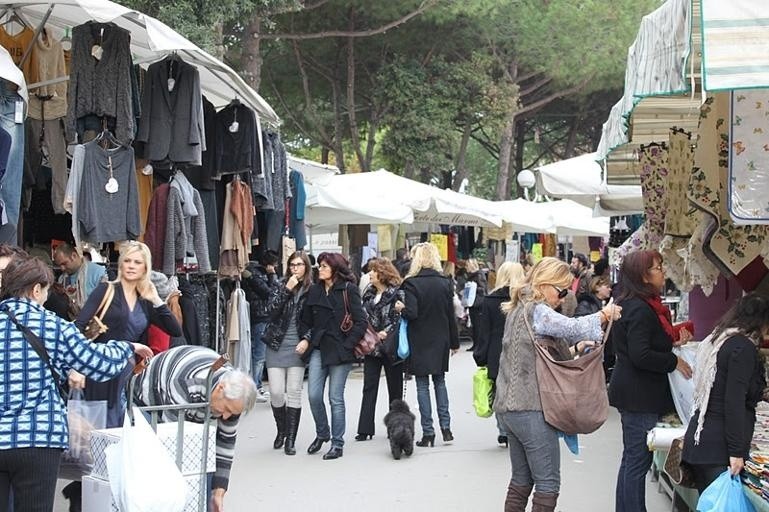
[285,406,301,455]
[271,401,286,449]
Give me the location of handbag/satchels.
[397,318,410,360]
[72,315,108,341]
[534,338,609,434]
[340,313,381,359]
[379,321,402,366]
[663,436,697,488]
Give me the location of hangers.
[1,10,25,29]
[61,27,72,43]
[166,50,182,65]
[97,119,123,145]
[227,94,243,110]
[95,132,118,146]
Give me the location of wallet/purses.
[672,321,694,342]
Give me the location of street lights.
[516,169,535,200]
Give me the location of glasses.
[289,263,305,270]
[649,264,662,271]
[552,285,569,299]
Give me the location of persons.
[569,254,593,302]
[680,291,769,512]
[607,250,692,512]
[473,261,525,447]
[594,259,611,276]
[492,256,623,512]
[240,243,488,461]
[0,241,259,512]
[573,274,612,319]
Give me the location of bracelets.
[600,310,611,322]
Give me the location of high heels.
[416,435,436,447]
[498,434,508,446]
[355,434,372,440]
[442,427,452,441]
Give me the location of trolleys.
[82,353,231,512]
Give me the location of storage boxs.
[90,422,218,475]
[80,475,207,512]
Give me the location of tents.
[306,152,645,234]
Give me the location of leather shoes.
[324,448,342,460]
[308,436,330,454]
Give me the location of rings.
[682,337,687,344]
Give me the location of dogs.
[384,399,415,459]
